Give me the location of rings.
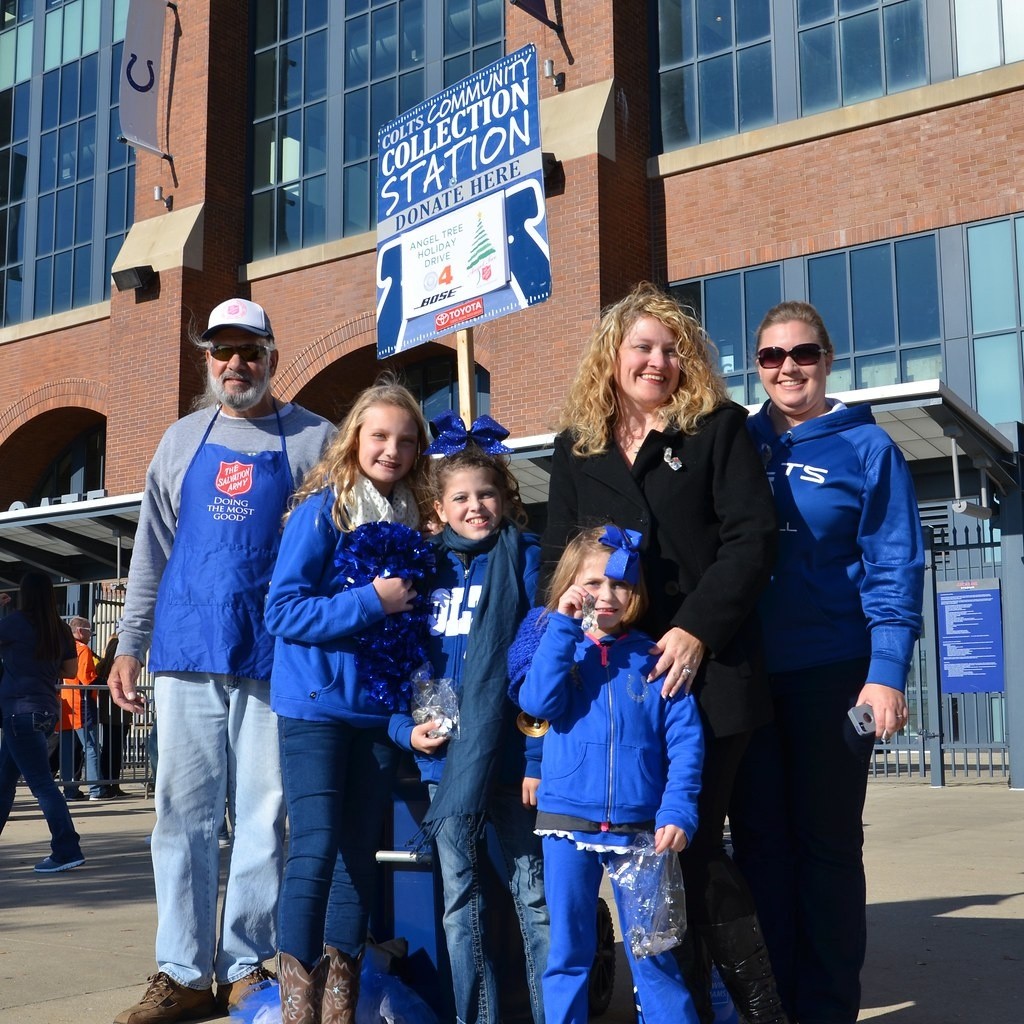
[683,667,692,676]
[896,714,903,718]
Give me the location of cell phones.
[847,704,877,736]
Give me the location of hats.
[202,298,275,344]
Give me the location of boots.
[274,951,330,1024]
[321,942,365,1024]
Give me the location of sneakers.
[217,970,272,1012]
[113,973,214,1023]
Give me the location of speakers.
[111,265,154,292]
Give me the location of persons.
[106,280,925,1023]
[45,617,133,801]
[0,569,88,872]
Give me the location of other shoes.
[115,788,134,797]
[88,792,118,799]
[34,847,86,872]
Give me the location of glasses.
[757,343,825,369]
[208,344,271,361]
[76,625,91,635]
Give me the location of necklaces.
[620,418,661,454]
